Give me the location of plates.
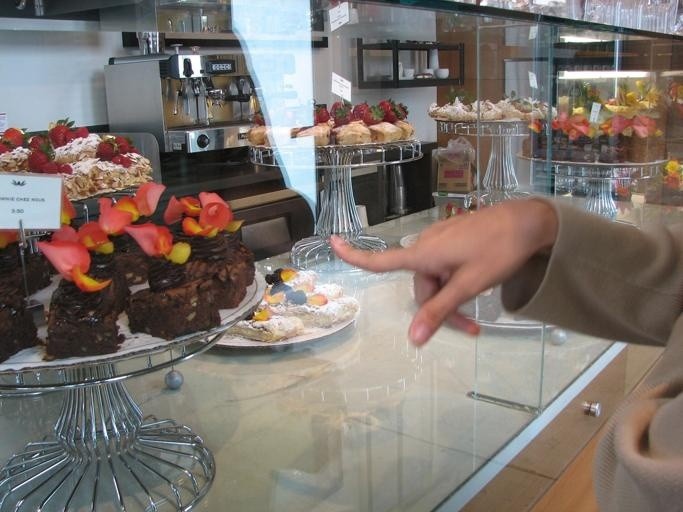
[463,309,558,332]
[388,76,415,81]
[214,271,357,349]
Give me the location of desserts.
[126,225,220,340]
[97,182,256,305]
[37,220,131,361]
[0,237,48,362]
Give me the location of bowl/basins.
[437,68,449,79]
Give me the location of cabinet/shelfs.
[356,36,464,89]
[0,0,683,512]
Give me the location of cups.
[424,69,433,74]
[404,69,415,77]
[582,1,678,33]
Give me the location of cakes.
[228,266,358,344]
[645,160,683,207]
[250,98,416,149]
[1,116,154,199]
[428,93,549,128]
[523,81,683,161]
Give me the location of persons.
[329,194,682,512]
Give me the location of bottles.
[398,56,403,78]
